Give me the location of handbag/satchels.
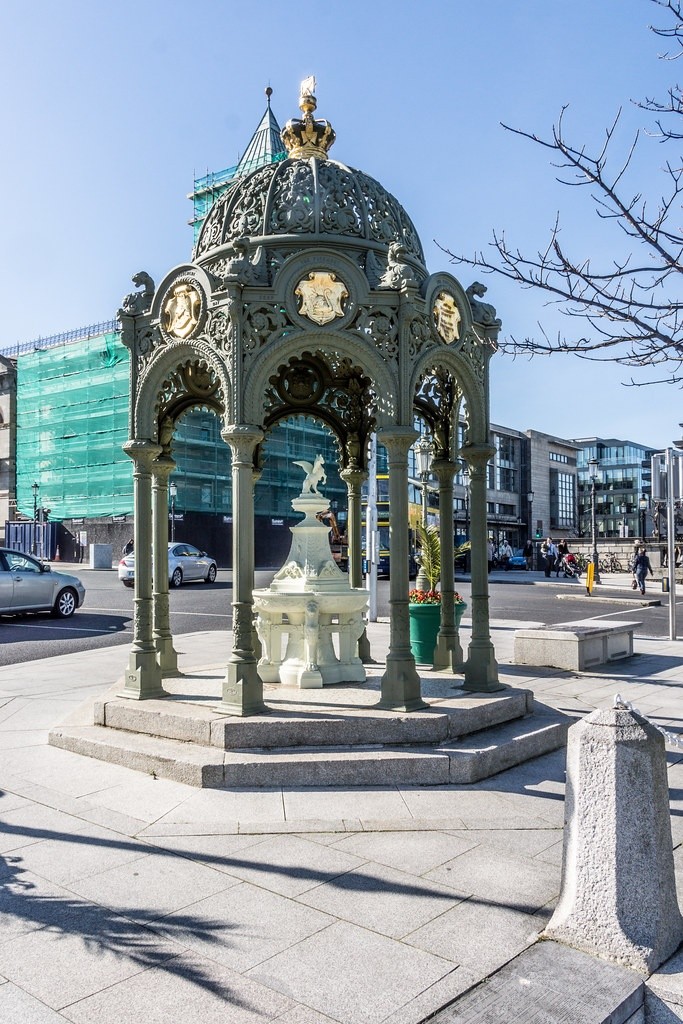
[630,573,637,590]
[501,554,508,562]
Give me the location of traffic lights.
[536,528,540,538]
[43,508,51,521]
[34,508,39,522]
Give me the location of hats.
[504,540,508,545]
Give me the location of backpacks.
[121,545,126,554]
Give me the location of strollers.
[558,553,582,578]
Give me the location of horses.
[301,454,327,493]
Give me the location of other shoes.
[545,575,552,578]
[556,575,560,578]
[640,588,645,595]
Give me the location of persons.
[487,538,496,574]
[125,538,134,556]
[501,541,513,571]
[632,548,653,595]
[540,537,569,578]
[525,540,533,570]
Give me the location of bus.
[348,475,440,579]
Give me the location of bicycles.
[574,552,623,573]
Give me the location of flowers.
[408,589,464,604]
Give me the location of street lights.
[527,490,535,540]
[413,431,434,591]
[621,503,627,537]
[460,471,471,542]
[169,481,177,543]
[31,482,40,556]
[639,495,648,543]
[586,457,601,581]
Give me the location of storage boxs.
[4,520,62,559]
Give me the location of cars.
[118,542,217,588]
[0,547,86,619]
[506,549,527,569]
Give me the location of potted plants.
[409,524,473,665]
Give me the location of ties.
[550,545,551,553]
